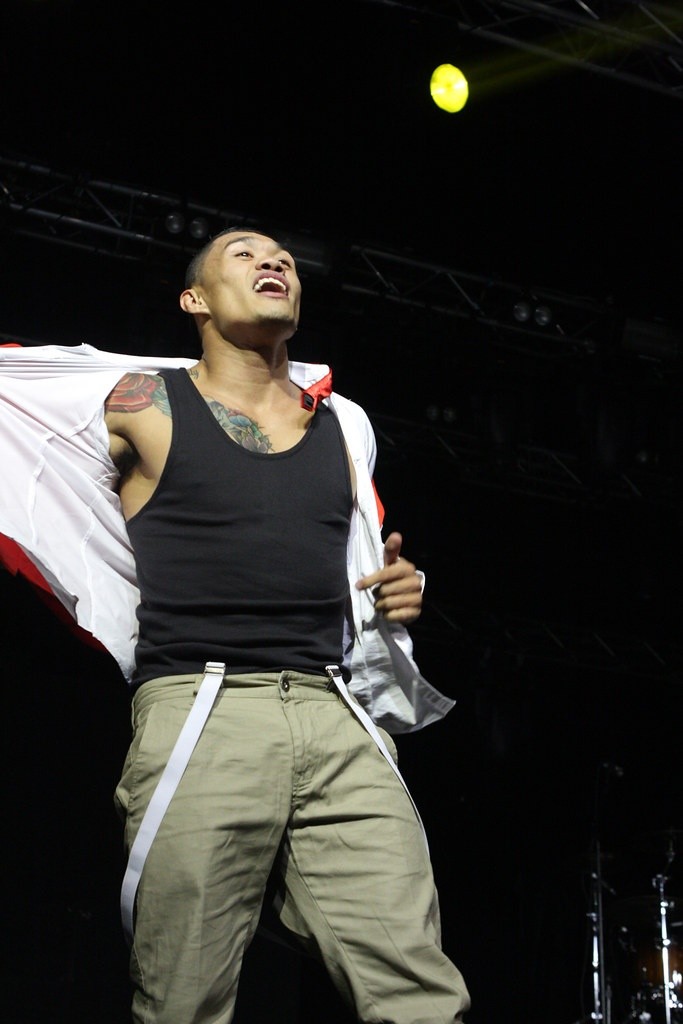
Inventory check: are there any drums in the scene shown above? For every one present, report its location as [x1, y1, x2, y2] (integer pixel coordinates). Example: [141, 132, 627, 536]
[626, 934, 683, 1003]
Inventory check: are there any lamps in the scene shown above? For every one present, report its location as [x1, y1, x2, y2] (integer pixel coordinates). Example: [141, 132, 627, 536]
[490, 287, 554, 331]
[159, 210, 210, 246]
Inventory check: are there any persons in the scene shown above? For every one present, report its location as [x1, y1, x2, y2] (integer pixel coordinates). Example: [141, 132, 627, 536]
[0, 228, 475, 1024]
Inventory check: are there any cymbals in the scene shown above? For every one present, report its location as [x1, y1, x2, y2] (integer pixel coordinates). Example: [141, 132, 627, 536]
[632, 829, 683, 854]
[609, 894, 683, 929]
